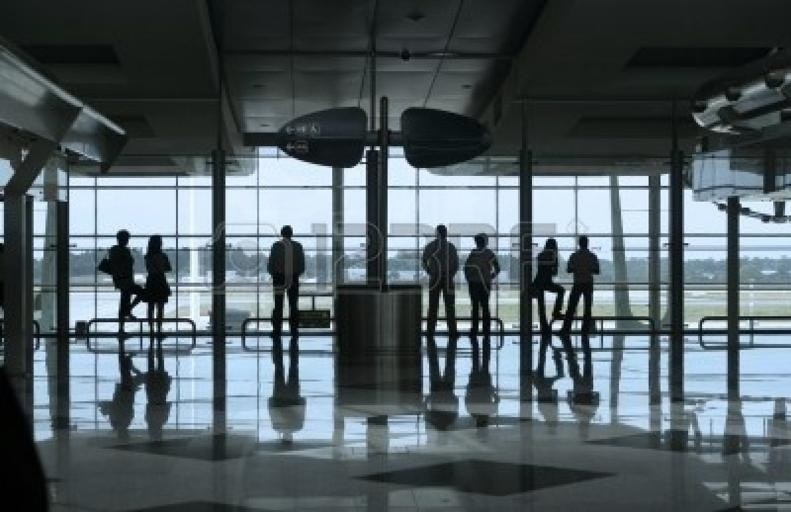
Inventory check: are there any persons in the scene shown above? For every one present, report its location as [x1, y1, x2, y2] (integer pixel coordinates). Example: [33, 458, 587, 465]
[142, 235, 173, 321]
[464, 334, 500, 427]
[533, 331, 564, 434]
[144, 321, 173, 444]
[562, 236, 599, 334]
[422, 225, 459, 336]
[97, 319, 144, 442]
[423, 336, 459, 430]
[537, 239, 565, 331]
[559, 333, 599, 442]
[464, 233, 501, 334]
[267, 225, 306, 336]
[268, 336, 306, 446]
[108, 231, 143, 321]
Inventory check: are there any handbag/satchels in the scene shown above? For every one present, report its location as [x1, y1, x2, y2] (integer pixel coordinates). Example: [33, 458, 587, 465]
[98, 249, 119, 276]
